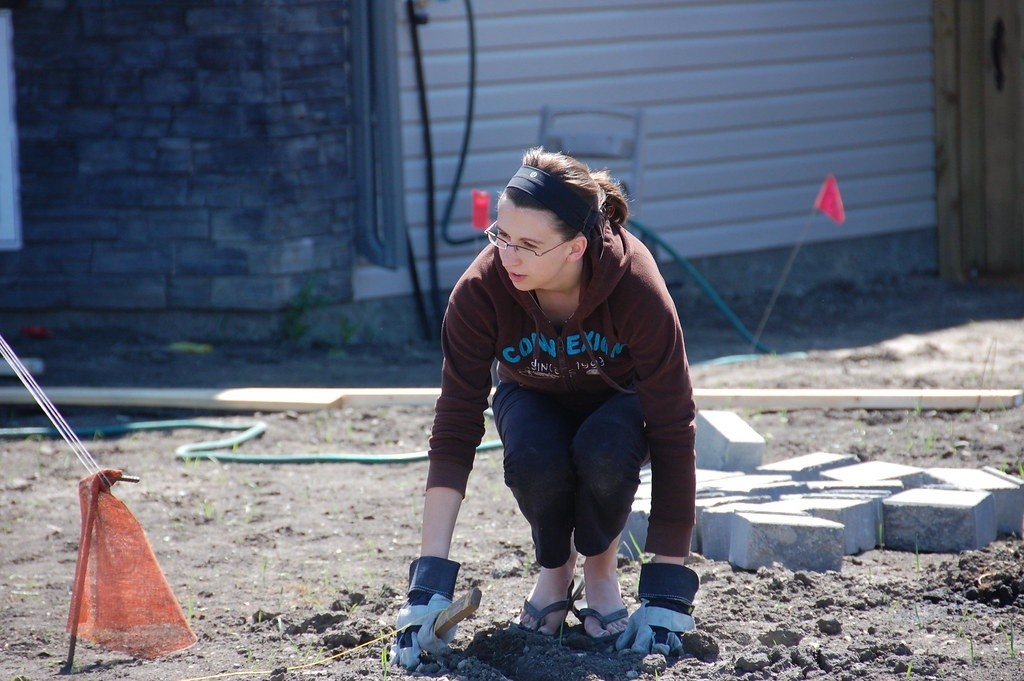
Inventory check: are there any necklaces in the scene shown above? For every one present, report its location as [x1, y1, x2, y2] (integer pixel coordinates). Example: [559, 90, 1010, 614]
[528, 291, 574, 323]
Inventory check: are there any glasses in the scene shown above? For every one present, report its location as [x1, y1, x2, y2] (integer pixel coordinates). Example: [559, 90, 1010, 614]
[484, 220, 571, 259]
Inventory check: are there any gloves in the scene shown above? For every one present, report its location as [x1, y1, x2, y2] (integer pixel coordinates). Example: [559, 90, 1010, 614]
[615, 563, 700, 660]
[389, 556, 461, 671]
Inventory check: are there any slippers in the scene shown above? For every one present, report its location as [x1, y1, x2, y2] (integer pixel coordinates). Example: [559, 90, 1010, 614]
[520, 579, 574, 639]
[578, 607, 628, 643]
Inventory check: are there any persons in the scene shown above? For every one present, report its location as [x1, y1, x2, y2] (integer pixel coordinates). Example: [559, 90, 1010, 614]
[389, 147, 699, 669]
